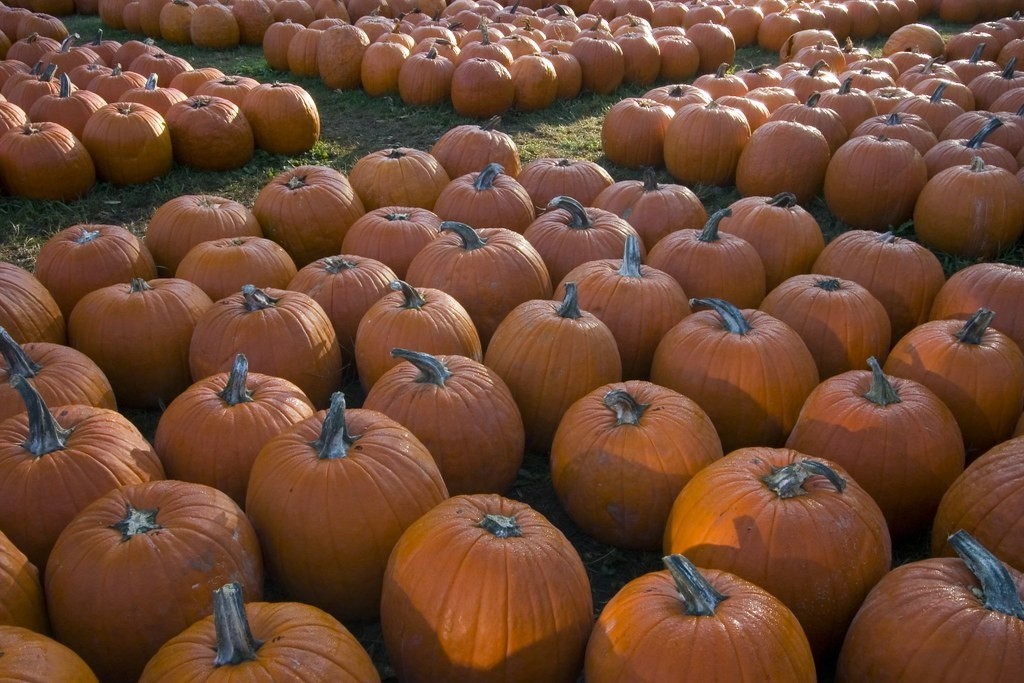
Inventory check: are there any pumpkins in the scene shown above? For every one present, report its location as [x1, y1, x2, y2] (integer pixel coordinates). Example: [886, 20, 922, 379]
[0, 113, 1024, 683]
[0, 0, 1024, 257]
